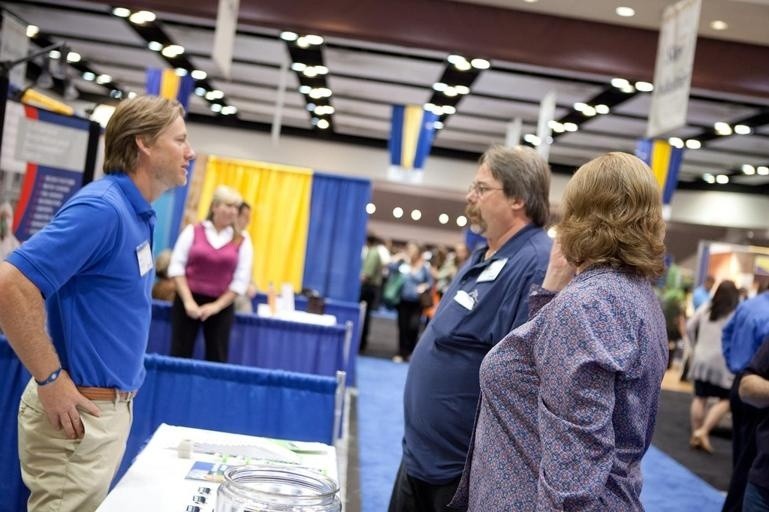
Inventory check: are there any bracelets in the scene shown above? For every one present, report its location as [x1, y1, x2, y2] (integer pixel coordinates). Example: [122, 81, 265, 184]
[34, 365, 63, 386]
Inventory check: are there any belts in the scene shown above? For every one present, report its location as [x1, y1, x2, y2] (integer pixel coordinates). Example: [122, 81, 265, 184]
[79, 387, 137, 402]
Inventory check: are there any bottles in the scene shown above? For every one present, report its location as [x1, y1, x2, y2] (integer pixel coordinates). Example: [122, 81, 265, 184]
[216, 460, 343, 511]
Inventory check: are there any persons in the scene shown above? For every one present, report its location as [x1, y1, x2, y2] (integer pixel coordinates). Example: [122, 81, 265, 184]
[358, 233, 469, 363]
[236, 202, 251, 232]
[0, 201, 21, 263]
[661, 267, 769, 512]
[167, 184, 254, 364]
[446, 152, 669, 511]
[387, 144, 554, 512]
[151, 247, 177, 303]
[0, 94, 196, 512]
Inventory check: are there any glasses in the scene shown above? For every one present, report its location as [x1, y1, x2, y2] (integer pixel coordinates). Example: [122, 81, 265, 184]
[467, 183, 504, 197]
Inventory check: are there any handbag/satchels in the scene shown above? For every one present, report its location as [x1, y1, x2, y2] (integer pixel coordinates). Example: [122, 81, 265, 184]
[383, 275, 404, 310]
[421, 288, 434, 307]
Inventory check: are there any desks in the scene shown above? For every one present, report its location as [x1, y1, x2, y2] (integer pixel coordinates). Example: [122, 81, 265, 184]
[94, 421, 343, 512]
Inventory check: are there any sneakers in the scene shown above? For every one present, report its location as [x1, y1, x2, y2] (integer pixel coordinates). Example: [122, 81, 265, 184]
[691, 429, 712, 455]
